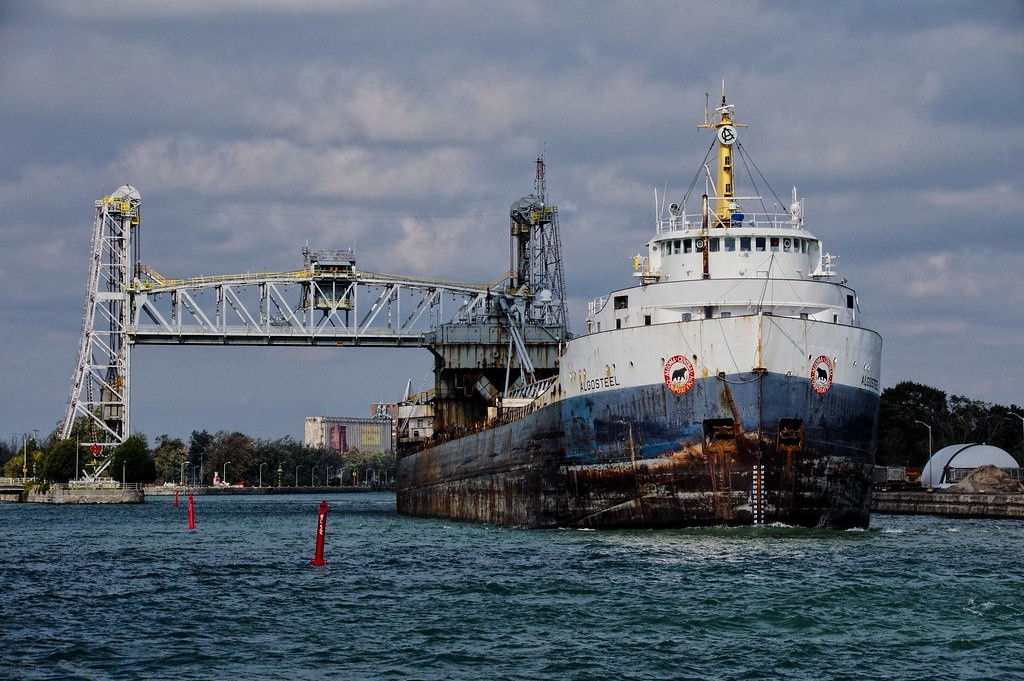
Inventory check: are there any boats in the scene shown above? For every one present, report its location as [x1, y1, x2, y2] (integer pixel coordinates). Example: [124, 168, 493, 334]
[395, 78, 882, 540]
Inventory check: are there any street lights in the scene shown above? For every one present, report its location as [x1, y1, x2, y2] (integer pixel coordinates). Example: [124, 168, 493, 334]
[259, 463, 267, 487]
[326, 466, 332, 486]
[224, 462, 230, 483]
[194, 466, 200, 486]
[295, 465, 302, 487]
[180, 461, 190, 488]
[312, 466, 318, 487]
[353, 468, 359, 486]
[915, 420, 933, 489]
[340, 467, 346, 486]
[366, 468, 387, 486]
